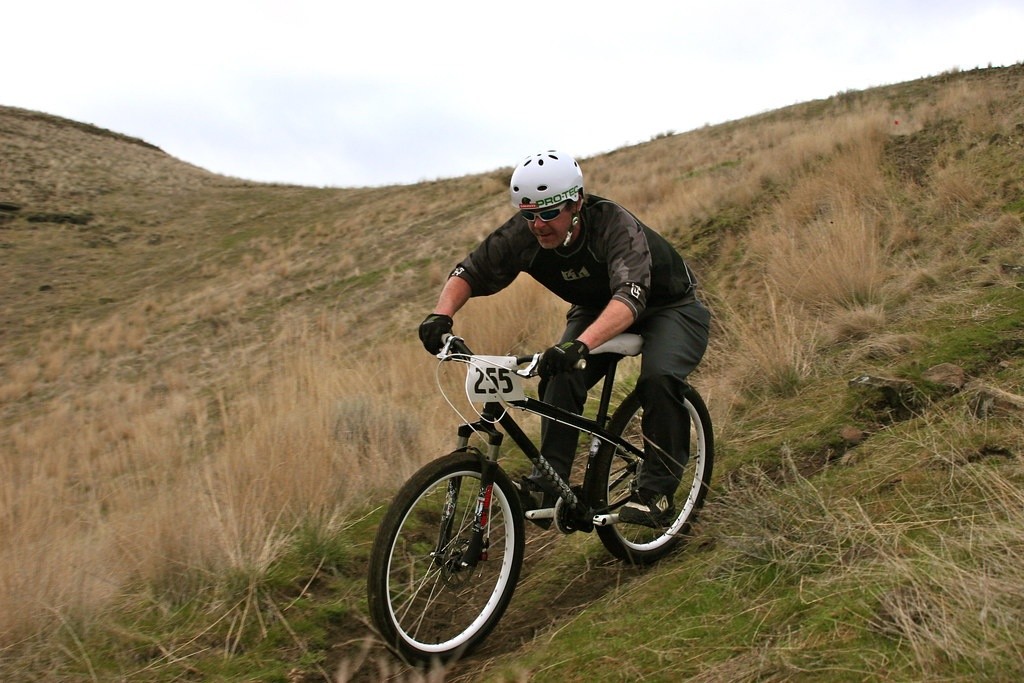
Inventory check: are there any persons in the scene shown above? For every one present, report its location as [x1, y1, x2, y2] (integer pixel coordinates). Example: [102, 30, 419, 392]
[420, 148, 711, 531]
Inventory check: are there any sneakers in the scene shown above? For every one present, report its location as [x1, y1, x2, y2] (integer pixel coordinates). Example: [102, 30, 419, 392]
[618, 489, 677, 528]
[511, 477, 557, 530]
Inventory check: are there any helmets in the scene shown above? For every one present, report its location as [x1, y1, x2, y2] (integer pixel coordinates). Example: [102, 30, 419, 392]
[509, 149, 585, 209]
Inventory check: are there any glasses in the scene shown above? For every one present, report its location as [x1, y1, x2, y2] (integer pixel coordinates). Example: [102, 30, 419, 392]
[519, 200, 569, 221]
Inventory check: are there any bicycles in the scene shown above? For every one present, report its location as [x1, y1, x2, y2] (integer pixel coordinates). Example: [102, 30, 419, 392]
[365, 326, 714, 666]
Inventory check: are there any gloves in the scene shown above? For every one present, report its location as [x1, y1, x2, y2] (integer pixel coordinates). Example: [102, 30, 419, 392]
[537, 338, 589, 382]
[418, 313, 454, 355]
